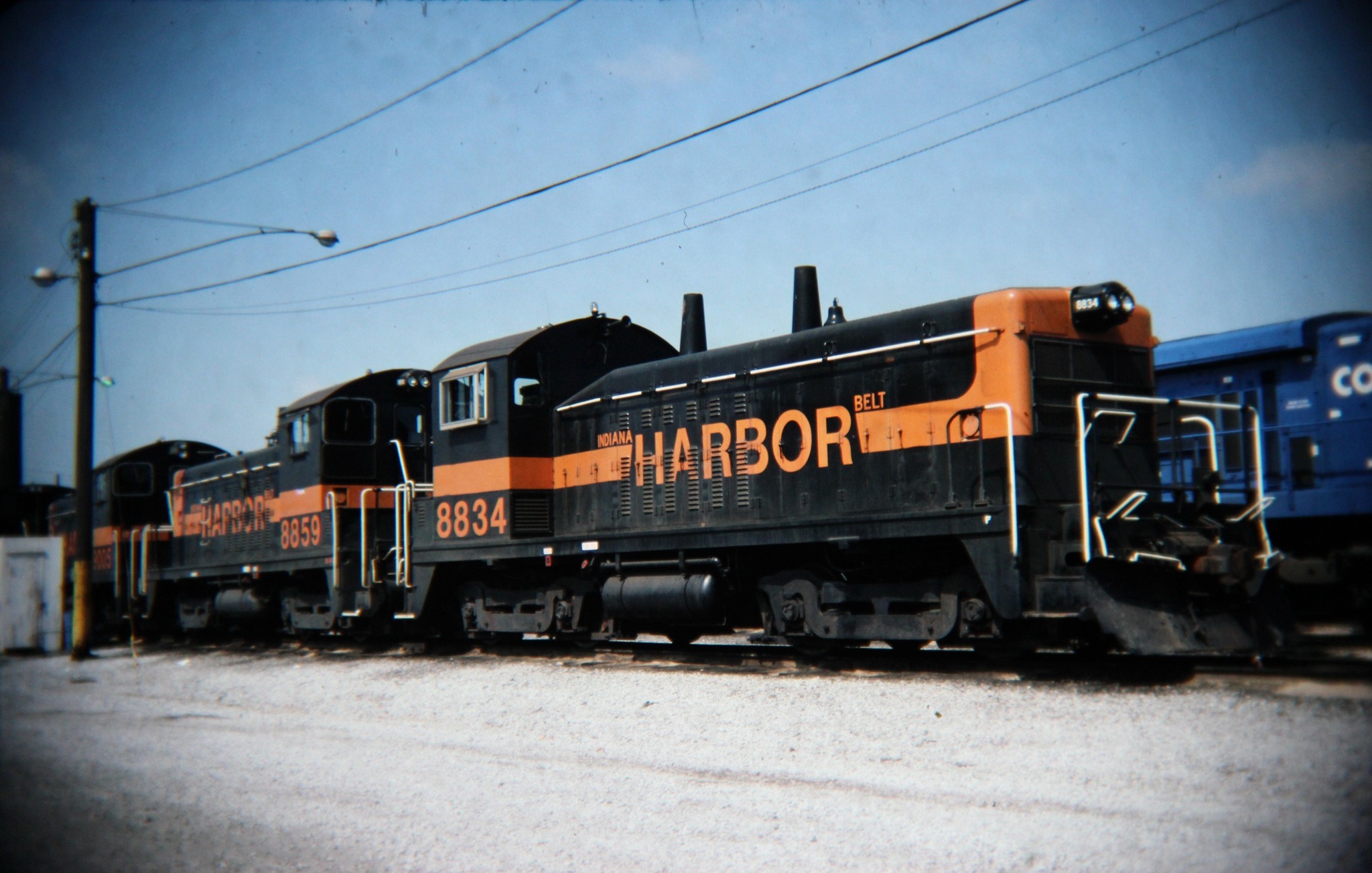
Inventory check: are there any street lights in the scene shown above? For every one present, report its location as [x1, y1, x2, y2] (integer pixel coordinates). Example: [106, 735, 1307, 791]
[31, 198, 344, 664]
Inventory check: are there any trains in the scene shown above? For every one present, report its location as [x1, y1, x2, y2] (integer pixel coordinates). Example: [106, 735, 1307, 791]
[1151, 308, 1372, 623]
[46, 264, 1307, 683]
